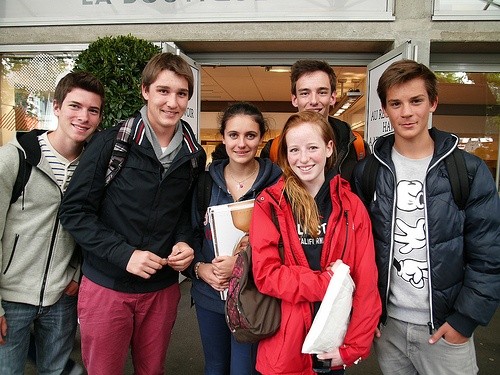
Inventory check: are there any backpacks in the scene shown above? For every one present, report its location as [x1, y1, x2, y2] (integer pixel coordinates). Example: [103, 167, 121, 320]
[224, 244, 280, 343]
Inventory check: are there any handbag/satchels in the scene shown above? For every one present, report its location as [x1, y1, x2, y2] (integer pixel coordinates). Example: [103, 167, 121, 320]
[301, 259, 361, 364]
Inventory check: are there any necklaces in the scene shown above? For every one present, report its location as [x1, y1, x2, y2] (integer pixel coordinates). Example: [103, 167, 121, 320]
[228, 160, 257, 189]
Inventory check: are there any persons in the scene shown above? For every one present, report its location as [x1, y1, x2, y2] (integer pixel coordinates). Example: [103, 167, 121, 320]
[178, 102, 284, 375]
[260, 58, 372, 186]
[0, 73, 105, 375]
[249, 108, 383, 375]
[58, 52, 208, 375]
[349, 61, 500, 374]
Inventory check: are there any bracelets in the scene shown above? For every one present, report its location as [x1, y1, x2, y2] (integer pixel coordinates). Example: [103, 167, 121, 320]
[195, 262, 204, 279]
